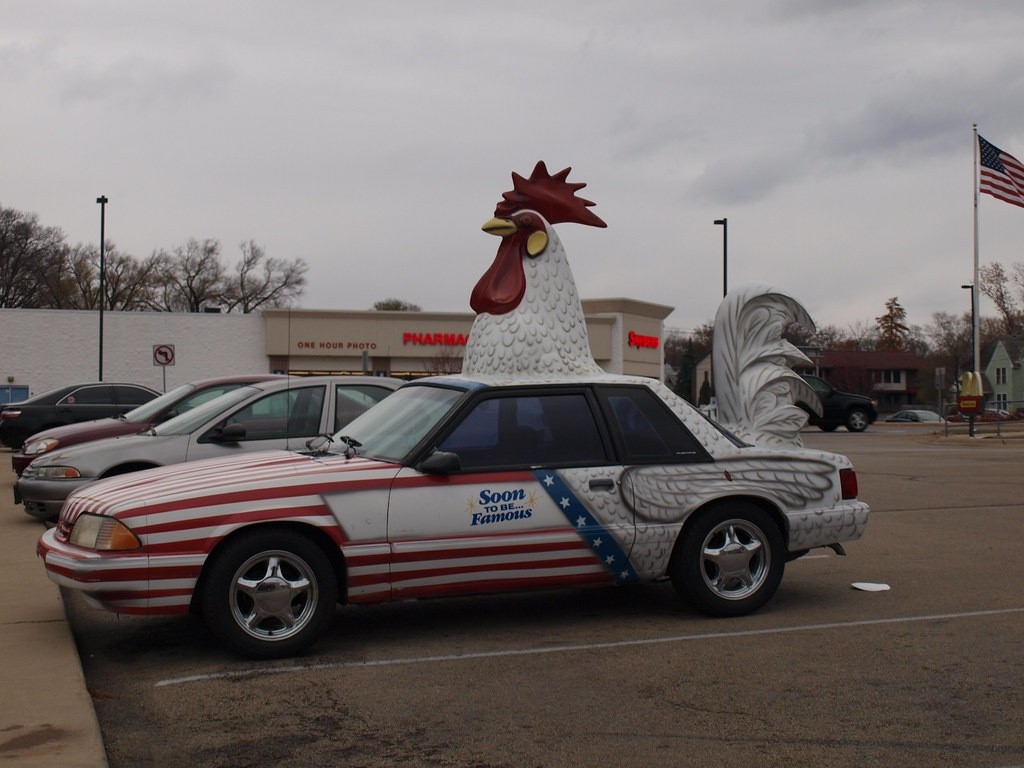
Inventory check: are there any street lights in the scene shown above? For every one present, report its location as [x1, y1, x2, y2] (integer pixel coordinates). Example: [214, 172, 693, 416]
[961, 284, 976, 372]
[95, 193, 110, 381]
[712, 217, 728, 299]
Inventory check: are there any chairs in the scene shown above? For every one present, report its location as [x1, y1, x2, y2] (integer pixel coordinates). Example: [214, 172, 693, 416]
[556, 425, 604, 461]
[483, 426, 535, 469]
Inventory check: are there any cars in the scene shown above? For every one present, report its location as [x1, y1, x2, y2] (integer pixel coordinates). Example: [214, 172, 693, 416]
[9, 374, 456, 525]
[883, 409, 947, 423]
[946, 405, 1024, 424]
[0, 380, 196, 452]
[31, 160, 871, 665]
[10, 373, 370, 476]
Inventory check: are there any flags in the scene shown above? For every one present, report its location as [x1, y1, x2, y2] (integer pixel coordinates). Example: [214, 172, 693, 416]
[978, 135, 1024, 208]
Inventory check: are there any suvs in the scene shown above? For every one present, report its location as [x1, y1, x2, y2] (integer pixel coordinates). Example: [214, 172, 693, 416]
[795, 373, 880, 433]
